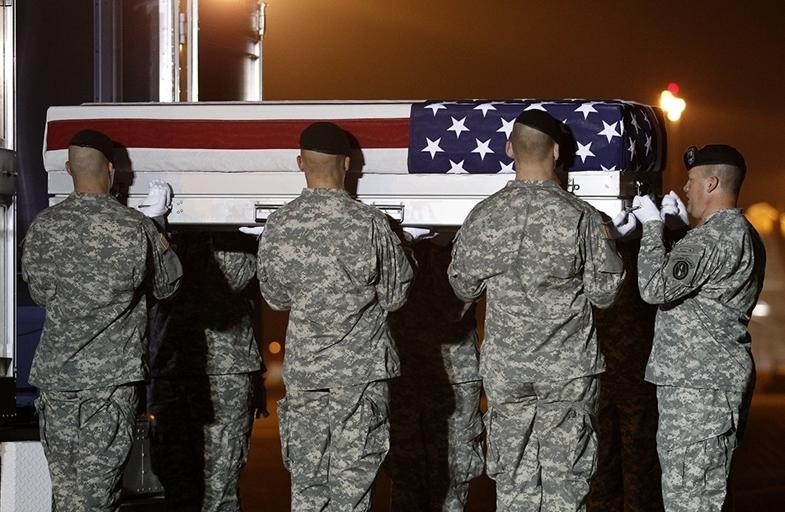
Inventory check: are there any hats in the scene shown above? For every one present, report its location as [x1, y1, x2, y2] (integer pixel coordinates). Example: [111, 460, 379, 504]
[514, 110, 573, 147]
[299, 123, 354, 157]
[69, 130, 117, 169]
[684, 144, 747, 172]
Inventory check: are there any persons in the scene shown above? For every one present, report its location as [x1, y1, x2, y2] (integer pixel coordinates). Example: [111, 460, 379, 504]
[255, 120, 421, 511]
[372, 225, 486, 511]
[147, 219, 266, 511]
[20, 129, 185, 512]
[444, 108, 637, 510]
[632, 132, 768, 511]
[584, 225, 687, 510]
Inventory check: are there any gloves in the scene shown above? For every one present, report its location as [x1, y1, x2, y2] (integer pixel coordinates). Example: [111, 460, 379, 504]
[660, 190, 690, 231]
[137, 180, 172, 219]
[608, 211, 637, 242]
[631, 194, 663, 225]
